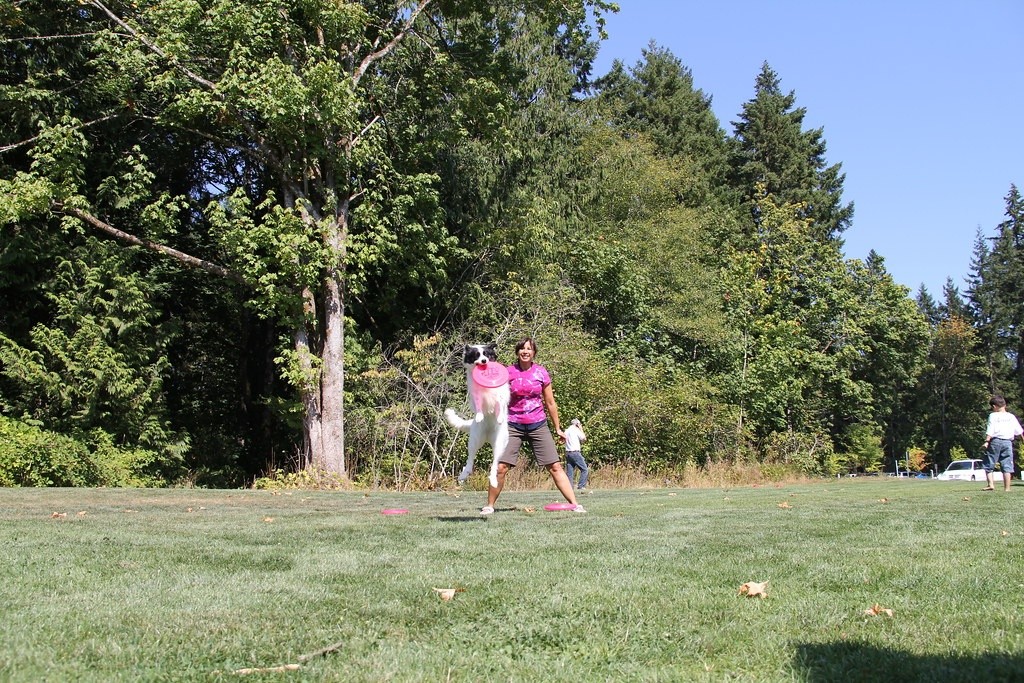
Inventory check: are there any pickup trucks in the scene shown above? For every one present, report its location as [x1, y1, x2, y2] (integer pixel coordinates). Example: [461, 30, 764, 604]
[938, 460, 1004, 482]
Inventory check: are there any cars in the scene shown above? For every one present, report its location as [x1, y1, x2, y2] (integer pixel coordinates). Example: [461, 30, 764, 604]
[888, 472, 931, 479]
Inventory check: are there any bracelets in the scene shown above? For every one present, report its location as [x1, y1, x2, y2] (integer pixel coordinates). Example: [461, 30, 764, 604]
[984, 440, 989, 443]
[556, 430, 561, 433]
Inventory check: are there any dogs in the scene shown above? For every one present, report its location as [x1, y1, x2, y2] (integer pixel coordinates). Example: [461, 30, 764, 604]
[443, 343, 511, 488]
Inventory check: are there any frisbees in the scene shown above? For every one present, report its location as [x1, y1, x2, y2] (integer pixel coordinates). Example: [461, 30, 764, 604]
[544, 502, 577, 511]
[471, 361, 511, 389]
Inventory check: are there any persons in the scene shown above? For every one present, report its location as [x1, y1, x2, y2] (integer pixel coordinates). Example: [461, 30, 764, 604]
[979, 395, 1024, 491]
[564, 418, 578, 489]
[481, 338, 586, 515]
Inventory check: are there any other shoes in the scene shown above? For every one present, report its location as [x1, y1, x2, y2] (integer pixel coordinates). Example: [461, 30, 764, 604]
[480, 506, 494, 514]
[573, 503, 586, 513]
